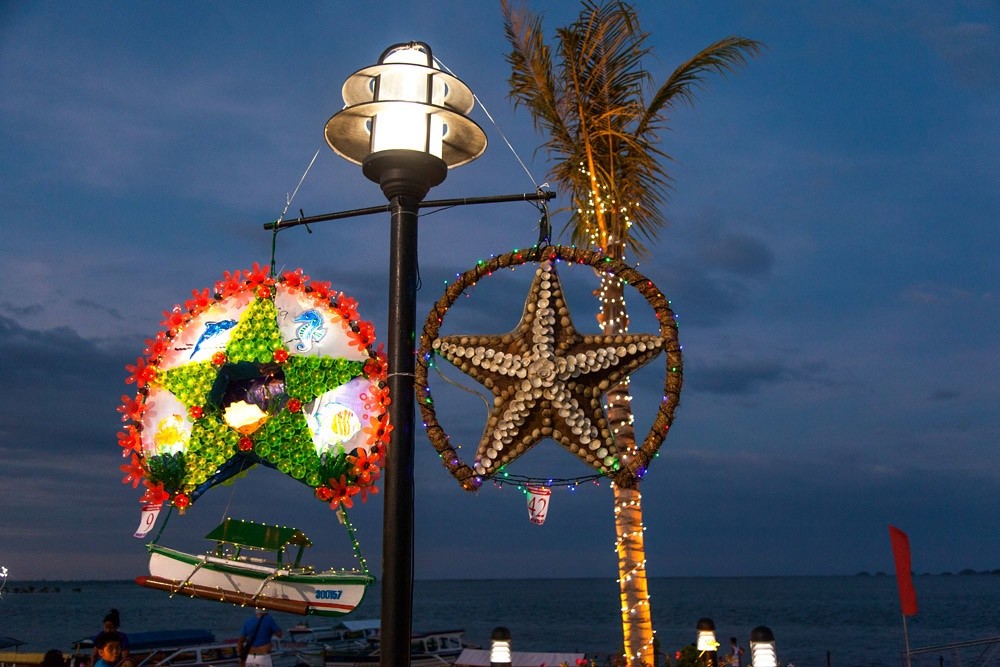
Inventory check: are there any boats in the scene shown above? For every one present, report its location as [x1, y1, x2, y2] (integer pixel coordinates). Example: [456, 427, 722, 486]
[135, 542, 378, 619]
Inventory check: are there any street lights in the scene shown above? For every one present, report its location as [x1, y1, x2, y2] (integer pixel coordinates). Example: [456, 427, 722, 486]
[263, 40, 559, 665]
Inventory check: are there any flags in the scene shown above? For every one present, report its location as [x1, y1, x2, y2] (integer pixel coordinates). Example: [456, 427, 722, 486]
[887, 524, 918, 618]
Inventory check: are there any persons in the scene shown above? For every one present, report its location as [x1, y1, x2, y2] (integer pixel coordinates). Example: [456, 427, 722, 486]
[94, 633, 121, 667]
[92, 609, 132, 666]
[238, 605, 283, 667]
[726, 637, 745, 667]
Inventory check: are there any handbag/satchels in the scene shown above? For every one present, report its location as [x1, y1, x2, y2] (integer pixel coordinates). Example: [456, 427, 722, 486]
[240, 640, 251, 660]
